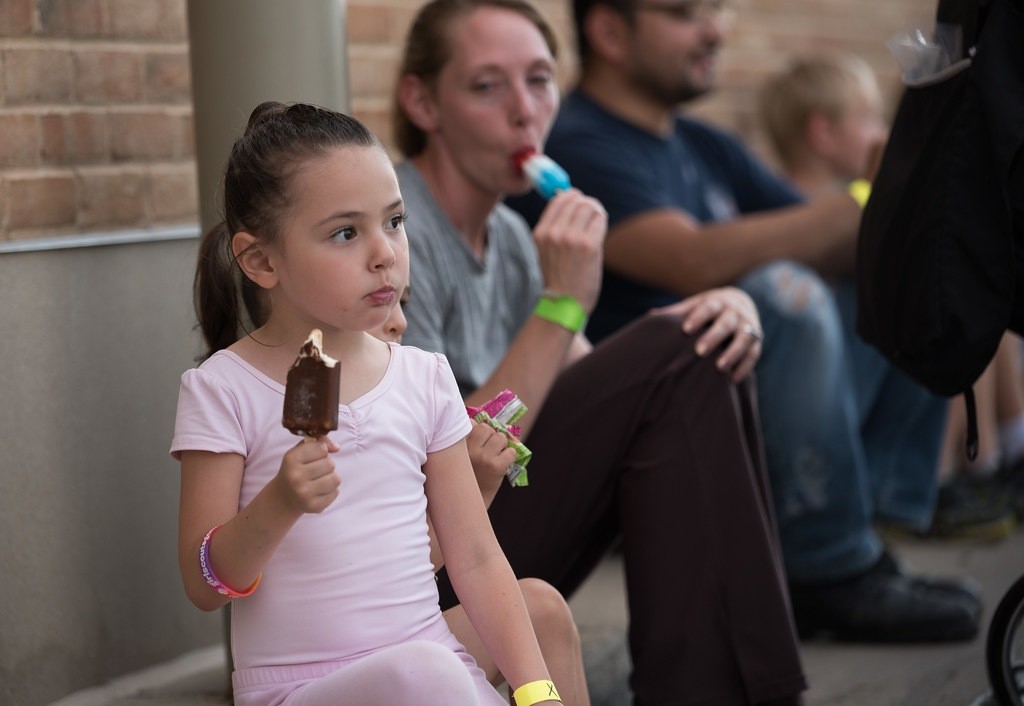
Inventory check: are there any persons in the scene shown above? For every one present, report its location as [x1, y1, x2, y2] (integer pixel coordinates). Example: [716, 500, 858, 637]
[760, 54, 1024, 539]
[167, 101, 565, 706]
[365, 267, 594, 706]
[503, 0, 985, 639]
[380, 0, 809, 706]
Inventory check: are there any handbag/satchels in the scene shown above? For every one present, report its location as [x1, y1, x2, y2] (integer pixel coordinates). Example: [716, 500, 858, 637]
[853, 0, 1021, 397]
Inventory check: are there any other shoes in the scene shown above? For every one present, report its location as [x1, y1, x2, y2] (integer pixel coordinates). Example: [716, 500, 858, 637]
[787, 554, 981, 645]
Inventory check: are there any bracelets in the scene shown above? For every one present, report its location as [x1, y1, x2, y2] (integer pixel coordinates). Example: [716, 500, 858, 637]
[535, 289, 588, 334]
[511, 680, 562, 706]
[197, 524, 263, 598]
